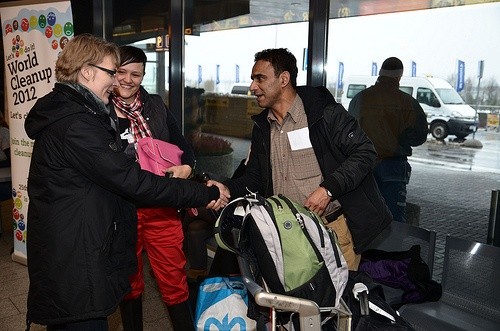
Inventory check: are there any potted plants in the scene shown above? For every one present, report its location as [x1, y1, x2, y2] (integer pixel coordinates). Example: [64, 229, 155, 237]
[192, 134, 236, 179]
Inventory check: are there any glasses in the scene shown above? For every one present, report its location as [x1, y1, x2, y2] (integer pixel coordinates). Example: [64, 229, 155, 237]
[88, 63, 117, 79]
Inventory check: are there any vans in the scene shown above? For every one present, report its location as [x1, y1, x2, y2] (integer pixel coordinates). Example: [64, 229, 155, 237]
[341, 74, 481, 141]
[216, 82, 257, 97]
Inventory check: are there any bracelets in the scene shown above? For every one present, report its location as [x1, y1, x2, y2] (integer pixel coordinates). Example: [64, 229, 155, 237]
[326, 189, 333, 198]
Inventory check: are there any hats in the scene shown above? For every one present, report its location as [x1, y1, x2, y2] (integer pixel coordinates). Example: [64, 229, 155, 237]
[379, 57, 403, 77]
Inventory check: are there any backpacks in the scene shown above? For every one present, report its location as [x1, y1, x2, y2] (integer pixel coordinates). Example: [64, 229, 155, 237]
[214, 193, 349, 331]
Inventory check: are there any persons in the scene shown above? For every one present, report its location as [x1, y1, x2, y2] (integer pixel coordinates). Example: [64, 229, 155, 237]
[25, 34, 229, 331]
[348, 57, 428, 223]
[108, 46, 197, 331]
[205, 47, 394, 271]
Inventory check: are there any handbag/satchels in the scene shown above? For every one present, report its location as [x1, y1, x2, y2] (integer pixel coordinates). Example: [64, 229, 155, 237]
[195, 277, 258, 331]
[137, 137, 184, 176]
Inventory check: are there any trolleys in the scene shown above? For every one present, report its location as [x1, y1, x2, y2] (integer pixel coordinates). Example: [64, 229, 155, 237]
[231, 221, 373, 331]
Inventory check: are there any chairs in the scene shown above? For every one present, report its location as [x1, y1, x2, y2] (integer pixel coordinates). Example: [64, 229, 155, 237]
[430, 92, 435, 106]
[421, 93, 430, 105]
[397, 236, 500, 331]
[361, 221, 437, 305]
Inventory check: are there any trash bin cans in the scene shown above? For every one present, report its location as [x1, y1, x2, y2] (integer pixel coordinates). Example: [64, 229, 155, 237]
[478, 113, 488, 129]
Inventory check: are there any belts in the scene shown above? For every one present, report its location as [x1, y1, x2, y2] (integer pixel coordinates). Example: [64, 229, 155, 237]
[321, 208, 342, 224]
